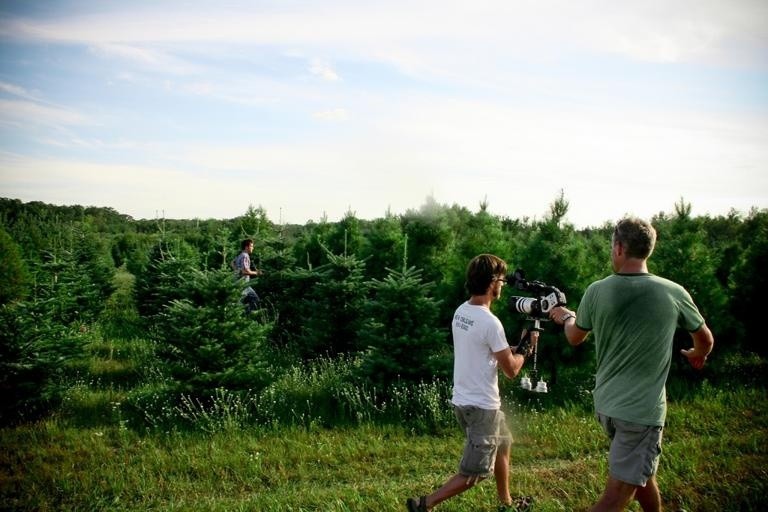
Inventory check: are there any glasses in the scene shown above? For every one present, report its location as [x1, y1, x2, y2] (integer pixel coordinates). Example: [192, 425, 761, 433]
[497, 278, 507, 285]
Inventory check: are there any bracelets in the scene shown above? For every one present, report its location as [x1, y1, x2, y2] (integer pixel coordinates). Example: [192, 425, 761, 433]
[560, 313, 576, 325]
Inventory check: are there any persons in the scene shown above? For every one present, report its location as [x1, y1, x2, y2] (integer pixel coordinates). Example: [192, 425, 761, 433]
[229, 239, 264, 317]
[544, 215, 715, 511]
[405, 251, 539, 512]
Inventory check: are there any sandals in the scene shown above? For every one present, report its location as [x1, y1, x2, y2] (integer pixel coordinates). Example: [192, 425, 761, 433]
[499, 496, 532, 512]
[407, 495, 427, 512]
